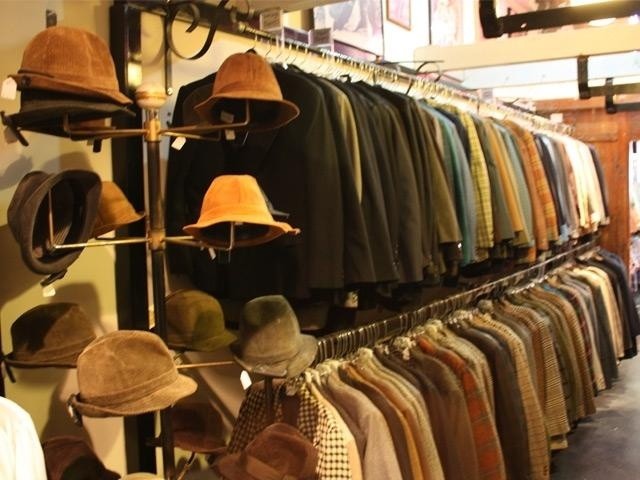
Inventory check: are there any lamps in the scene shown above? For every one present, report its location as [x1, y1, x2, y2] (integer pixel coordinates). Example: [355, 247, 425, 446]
[126, 58, 142, 93]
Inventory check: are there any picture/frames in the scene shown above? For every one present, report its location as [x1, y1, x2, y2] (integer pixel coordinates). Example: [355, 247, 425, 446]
[386, 0, 411, 31]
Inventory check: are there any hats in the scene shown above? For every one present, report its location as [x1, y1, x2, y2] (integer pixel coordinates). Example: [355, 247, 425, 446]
[41, 435, 121, 480]
[7, 169, 147, 274]
[0, 25, 136, 125]
[181, 174, 301, 248]
[192, 52, 301, 132]
[145, 401, 319, 480]
